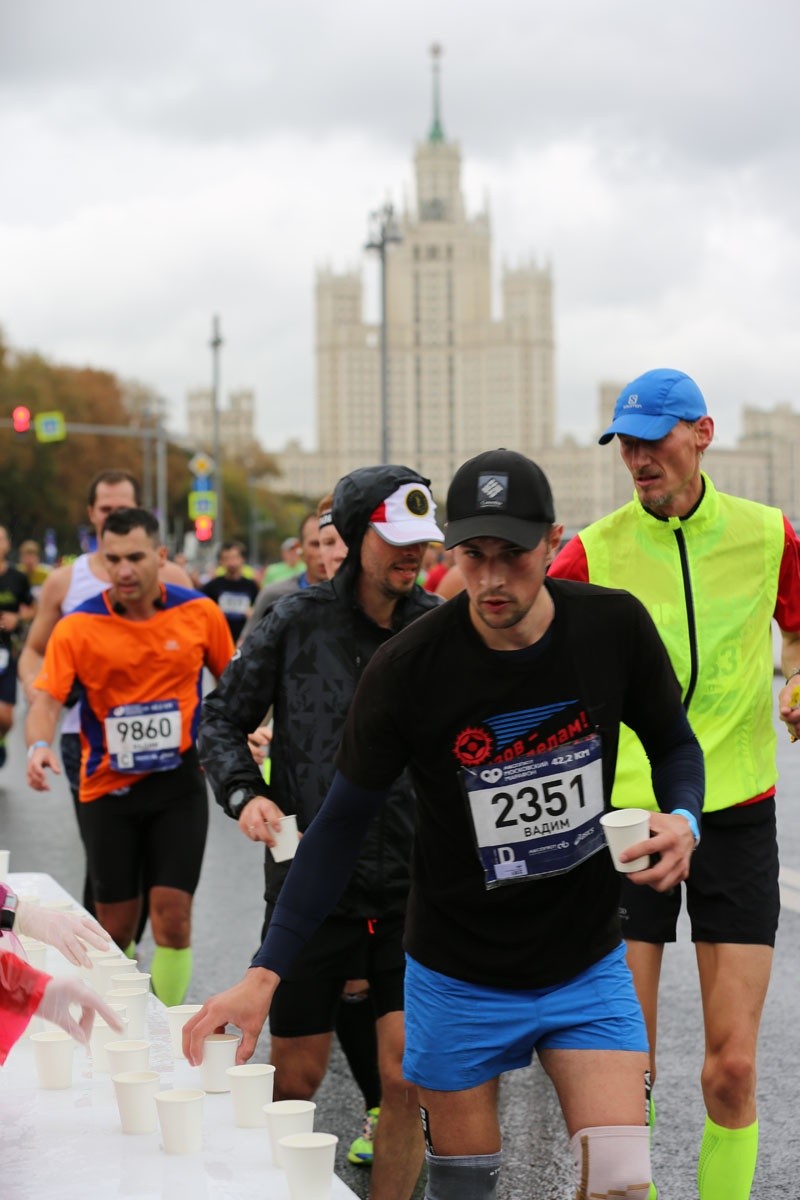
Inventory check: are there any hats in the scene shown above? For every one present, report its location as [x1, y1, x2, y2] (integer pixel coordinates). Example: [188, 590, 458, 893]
[368, 483, 445, 546]
[598, 368, 707, 445]
[444, 448, 555, 552]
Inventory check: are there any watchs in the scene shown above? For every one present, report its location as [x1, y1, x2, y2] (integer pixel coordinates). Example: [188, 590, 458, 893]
[228, 788, 258, 820]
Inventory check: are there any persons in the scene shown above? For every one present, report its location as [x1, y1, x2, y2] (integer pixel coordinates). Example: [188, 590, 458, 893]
[180, 448, 705, 1200]
[548, 368, 800, 1200]
[0, 469, 558, 1199]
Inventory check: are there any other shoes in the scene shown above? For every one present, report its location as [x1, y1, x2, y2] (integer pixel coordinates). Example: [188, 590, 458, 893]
[346, 1106, 381, 1164]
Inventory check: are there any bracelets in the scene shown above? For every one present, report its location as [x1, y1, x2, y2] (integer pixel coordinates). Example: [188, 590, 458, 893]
[786, 667, 799, 684]
[669, 809, 701, 848]
[28, 742, 49, 757]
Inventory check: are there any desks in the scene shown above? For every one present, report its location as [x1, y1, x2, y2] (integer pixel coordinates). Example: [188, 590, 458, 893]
[1, 873, 359, 1200]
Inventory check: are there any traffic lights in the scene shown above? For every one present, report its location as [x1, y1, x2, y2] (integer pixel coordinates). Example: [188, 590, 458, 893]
[13, 407, 32, 449]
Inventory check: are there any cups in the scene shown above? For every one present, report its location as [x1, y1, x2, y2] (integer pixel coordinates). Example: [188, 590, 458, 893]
[228, 1063, 276, 1126]
[264, 815, 300, 863]
[95, 1004, 127, 1018]
[85, 951, 122, 989]
[167, 1004, 205, 1059]
[202, 1034, 240, 1093]
[155, 1089, 207, 1153]
[97, 959, 138, 996]
[31, 1031, 74, 1089]
[0, 850, 10, 883]
[263, 1100, 317, 1166]
[278, 1133, 339, 1200]
[21, 944, 48, 972]
[111, 973, 151, 993]
[600, 808, 651, 873]
[18, 895, 87, 943]
[104, 1040, 150, 1072]
[107, 988, 148, 1026]
[112, 1070, 161, 1135]
[90, 1017, 130, 1071]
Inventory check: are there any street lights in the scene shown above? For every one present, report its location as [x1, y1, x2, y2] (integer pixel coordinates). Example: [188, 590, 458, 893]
[359, 215, 407, 472]
